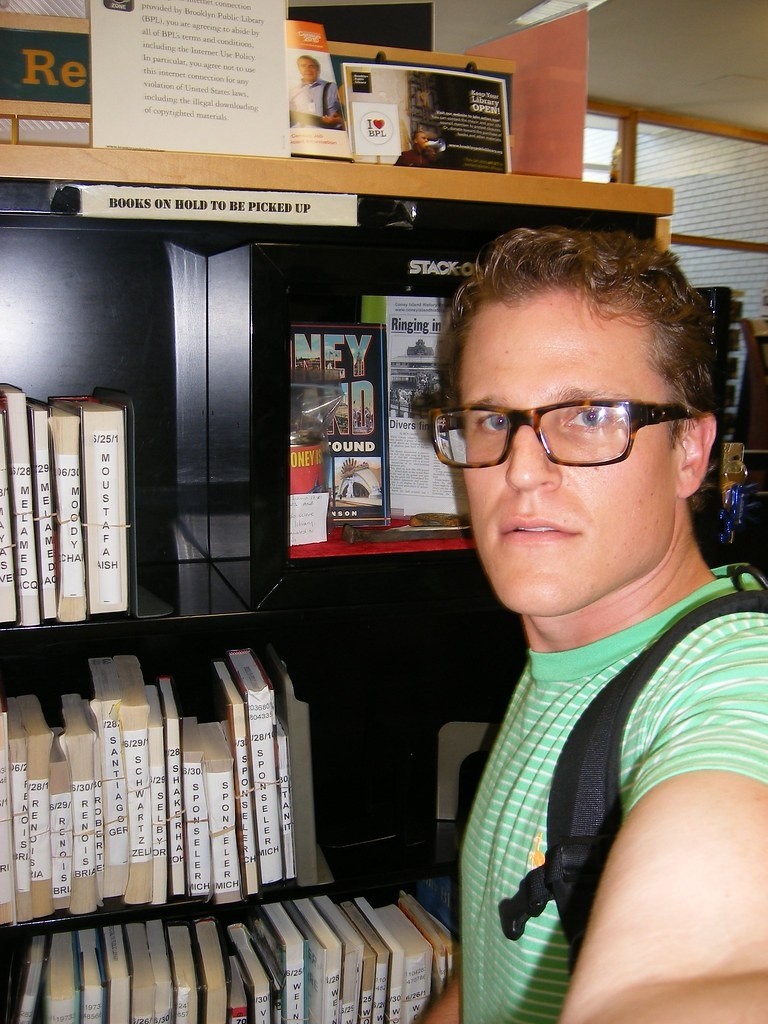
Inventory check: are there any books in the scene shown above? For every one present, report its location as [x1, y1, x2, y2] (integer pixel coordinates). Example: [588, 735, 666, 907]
[0, 0, 510, 1024]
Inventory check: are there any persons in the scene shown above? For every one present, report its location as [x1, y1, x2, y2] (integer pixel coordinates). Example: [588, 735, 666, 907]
[408, 226, 768, 1023]
[291, 55, 346, 130]
[394, 129, 442, 170]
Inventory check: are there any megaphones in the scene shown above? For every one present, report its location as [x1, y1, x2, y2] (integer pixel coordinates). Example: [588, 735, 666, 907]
[423, 138, 447, 152]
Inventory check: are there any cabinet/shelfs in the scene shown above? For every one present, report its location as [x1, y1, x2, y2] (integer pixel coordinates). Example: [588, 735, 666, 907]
[0, 139, 676, 1024]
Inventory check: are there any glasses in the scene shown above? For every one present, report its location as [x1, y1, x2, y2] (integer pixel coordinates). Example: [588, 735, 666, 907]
[428, 400, 694, 467]
